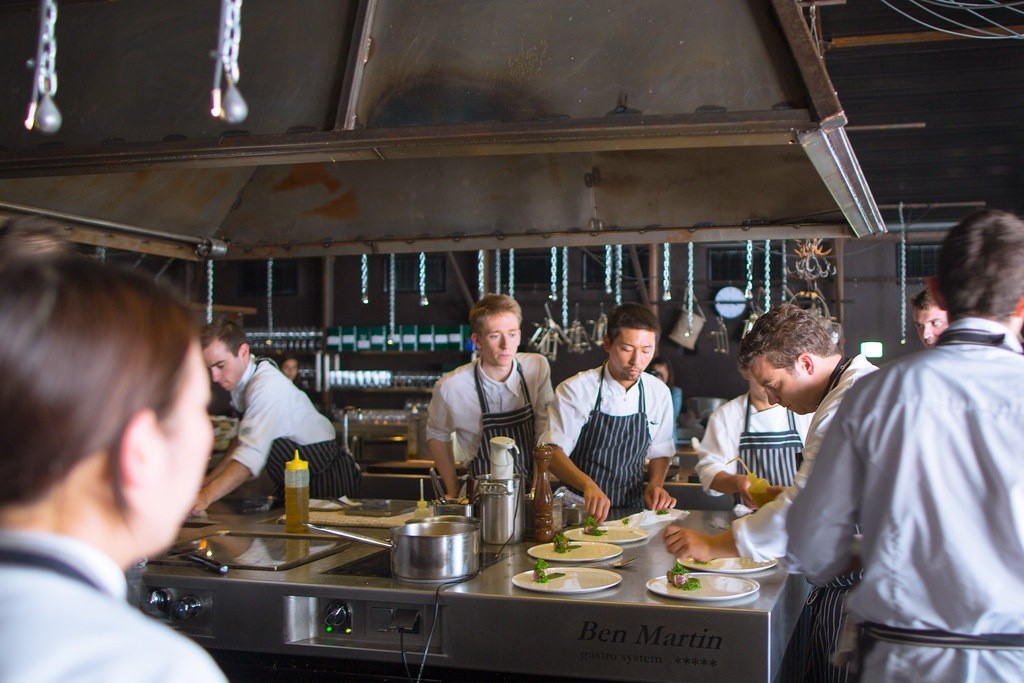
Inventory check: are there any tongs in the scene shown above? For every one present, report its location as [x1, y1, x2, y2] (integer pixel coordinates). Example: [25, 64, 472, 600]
[527, 302, 610, 362]
[429, 466, 447, 505]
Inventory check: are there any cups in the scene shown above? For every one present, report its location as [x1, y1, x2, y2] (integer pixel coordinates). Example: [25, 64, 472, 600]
[327, 368, 450, 427]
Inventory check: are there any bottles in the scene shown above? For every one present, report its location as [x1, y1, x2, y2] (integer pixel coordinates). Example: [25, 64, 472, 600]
[243, 326, 325, 352]
[284, 449, 311, 535]
[727, 458, 777, 510]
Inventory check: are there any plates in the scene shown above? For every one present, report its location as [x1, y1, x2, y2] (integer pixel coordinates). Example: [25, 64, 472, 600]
[511, 566, 623, 595]
[675, 555, 779, 574]
[345, 498, 419, 517]
[527, 540, 624, 562]
[563, 525, 648, 544]
[647, 571, 761, 602]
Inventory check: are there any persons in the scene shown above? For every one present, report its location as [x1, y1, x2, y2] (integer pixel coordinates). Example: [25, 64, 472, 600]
[647, 356, 683, 451]
[695, 341, 819, 511]
[535, 302, 680, 528]
[423, 294, 555, 502]
[181, 317, 368, 521]
[911, 288, 950, 350]
[661, 302, 883, 683]
[784, 211, 1023, 683]
[0, 270, 235, 683]
[279, 353, 303, 382]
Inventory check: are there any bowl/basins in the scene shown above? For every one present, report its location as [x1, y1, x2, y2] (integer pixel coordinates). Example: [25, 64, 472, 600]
[523, 493, 564, 534]
[685, 397, 730, 420]
[563, 503, 590, 526]
[433, 498, 475, 518]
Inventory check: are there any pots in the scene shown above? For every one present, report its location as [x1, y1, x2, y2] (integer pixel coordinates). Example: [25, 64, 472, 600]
[475, 473, 527, 545]
[298, 519, 483, 581]
[405, 513, 483, 562]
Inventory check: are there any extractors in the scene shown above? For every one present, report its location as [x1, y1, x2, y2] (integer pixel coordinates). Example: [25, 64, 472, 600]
[1, 0, 888, 262]
